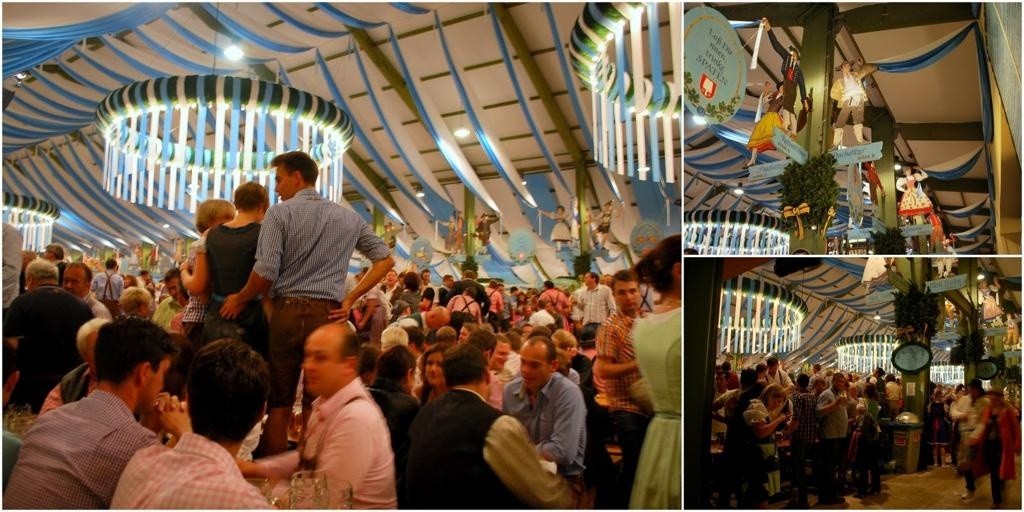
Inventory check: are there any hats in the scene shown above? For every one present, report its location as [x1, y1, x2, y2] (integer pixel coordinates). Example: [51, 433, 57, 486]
[987, 384, 1006, 398]
[579, 330, 595, 347]
[967, 379, 986, 393]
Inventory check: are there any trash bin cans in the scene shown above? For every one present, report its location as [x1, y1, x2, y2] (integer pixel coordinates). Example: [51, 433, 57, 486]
[888, 420, 924, 474]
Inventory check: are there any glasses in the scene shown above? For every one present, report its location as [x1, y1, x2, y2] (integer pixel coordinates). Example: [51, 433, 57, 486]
[24, 283, 31, 290]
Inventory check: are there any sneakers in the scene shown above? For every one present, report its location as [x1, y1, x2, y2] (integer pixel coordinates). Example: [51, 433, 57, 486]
[962, 493, 976, 503]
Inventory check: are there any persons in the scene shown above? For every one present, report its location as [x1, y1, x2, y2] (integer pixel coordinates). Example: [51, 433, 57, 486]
[829, 56, 878, 151]
[710, 355, 1021, 508]
[740, 80, 786, 170]
[864, 160, 886, 219]
[759, 17, 808, 141]
[587, 198, 622, 247]
[221, 152, 393, 456]
[434, 209, 503, 255]
[860, 257, 898, 296]
[924, 207, 946, 255]
[1003, 311, 1021, 350]
[895, 167, 933, 227]
[977, 275, 1003, 327]
[1, 222, 680, 508]
[937, 233, 958, 256]
[182, 199, 234, 347]
[179, 180, 269, 365]
[944, 297, 961, 327]
[537, 204, 573, 253]
[572, 194, 607, 251]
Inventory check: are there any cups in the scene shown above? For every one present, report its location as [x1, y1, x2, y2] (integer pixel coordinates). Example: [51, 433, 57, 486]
[248, 463, 356, 511]
